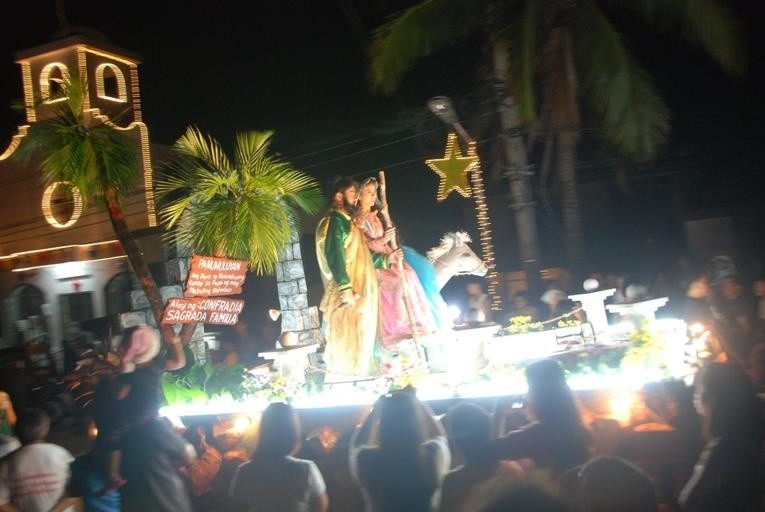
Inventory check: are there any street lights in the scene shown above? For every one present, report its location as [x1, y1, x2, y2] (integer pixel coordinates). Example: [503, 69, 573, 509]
[423, 92, 508, 315]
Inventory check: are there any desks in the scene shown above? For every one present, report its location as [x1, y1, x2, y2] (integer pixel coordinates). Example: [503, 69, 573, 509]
[568, 288, 619, 333]
[257, 342, 319, 389]
[604, 297, 669, 330]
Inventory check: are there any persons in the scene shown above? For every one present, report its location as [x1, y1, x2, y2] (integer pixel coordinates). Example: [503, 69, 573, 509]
[2, 268, 765, 512]
[352, 171, 436, 348]
[315, 175, 380, 392]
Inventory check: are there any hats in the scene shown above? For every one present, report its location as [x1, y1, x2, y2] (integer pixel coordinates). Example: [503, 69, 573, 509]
[119, 325, 160, 374]
